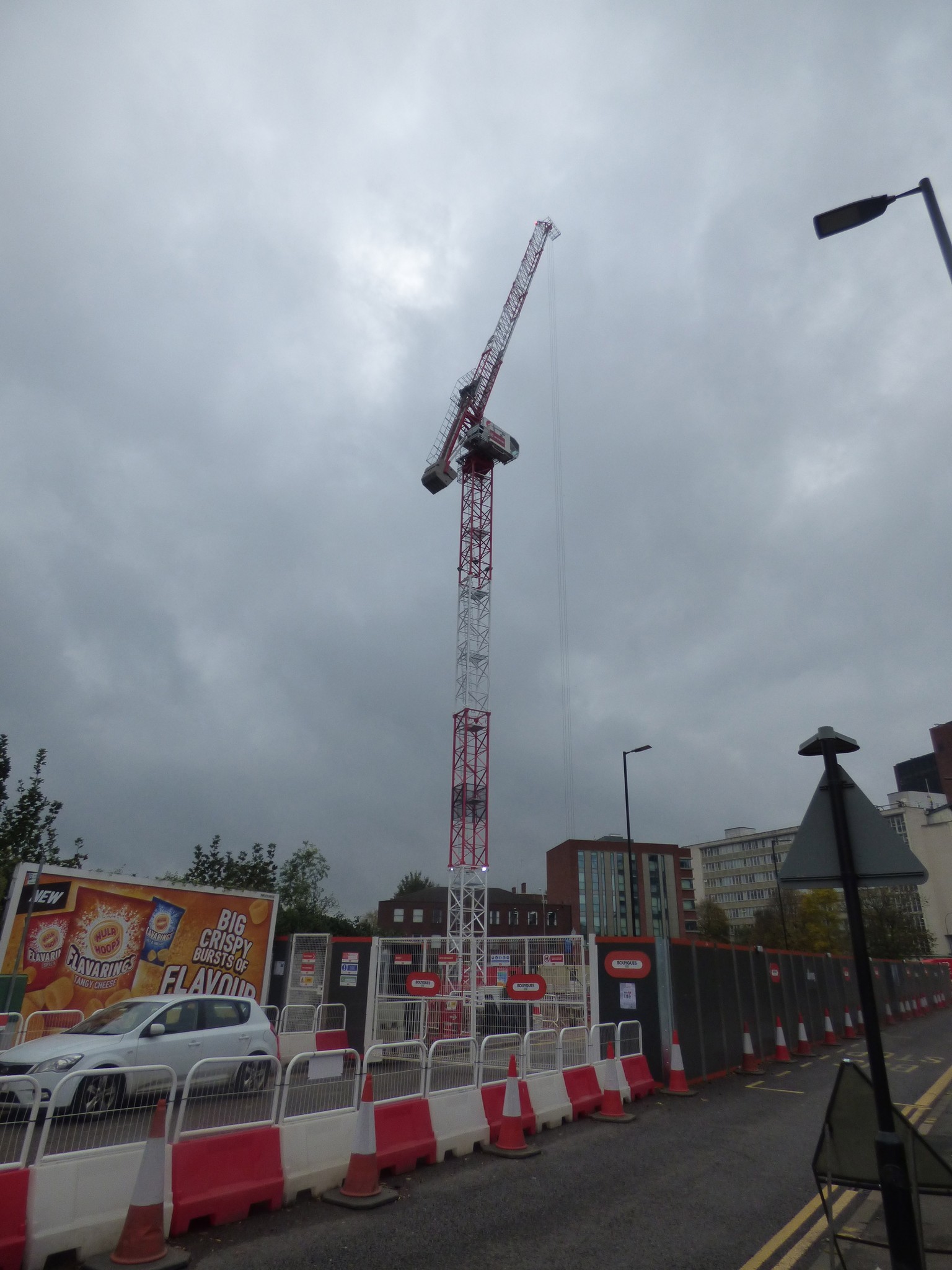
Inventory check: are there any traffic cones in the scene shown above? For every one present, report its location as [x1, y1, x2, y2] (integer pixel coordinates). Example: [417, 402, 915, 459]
[480, 1054, 544, 1160]
[659, 1030, 700, 1097]
[735, 1022, 771, 1076]
[80, 1099, 191, 1270]
[589, 1041, 637, 1123]
[318, 1074, 400, 1210]
[842, 990, 948, 1041]
[771, 1015, 796, 1063]
[821, 1007, 843, 1046]
[793, 1012, 818, 1057]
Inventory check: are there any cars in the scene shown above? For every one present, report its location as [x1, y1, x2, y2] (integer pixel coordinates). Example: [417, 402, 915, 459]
[0, 994, 280, 1120]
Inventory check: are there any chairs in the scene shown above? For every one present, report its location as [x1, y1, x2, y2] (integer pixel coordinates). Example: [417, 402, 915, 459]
[147, 1008, 167, 1029]
[176, 1007, 196, 1033]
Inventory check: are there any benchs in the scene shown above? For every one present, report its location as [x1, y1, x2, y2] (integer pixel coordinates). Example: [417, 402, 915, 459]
[204, 1012, 240, 1030]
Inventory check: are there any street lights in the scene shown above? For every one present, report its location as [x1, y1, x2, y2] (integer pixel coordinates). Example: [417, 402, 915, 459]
[770, 839, 794, 950]
[817, 176, 951, 278]
[622, 744, 654, 939]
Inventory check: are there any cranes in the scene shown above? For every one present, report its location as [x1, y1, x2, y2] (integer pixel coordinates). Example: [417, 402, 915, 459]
[422, 213, 561, 1000]
